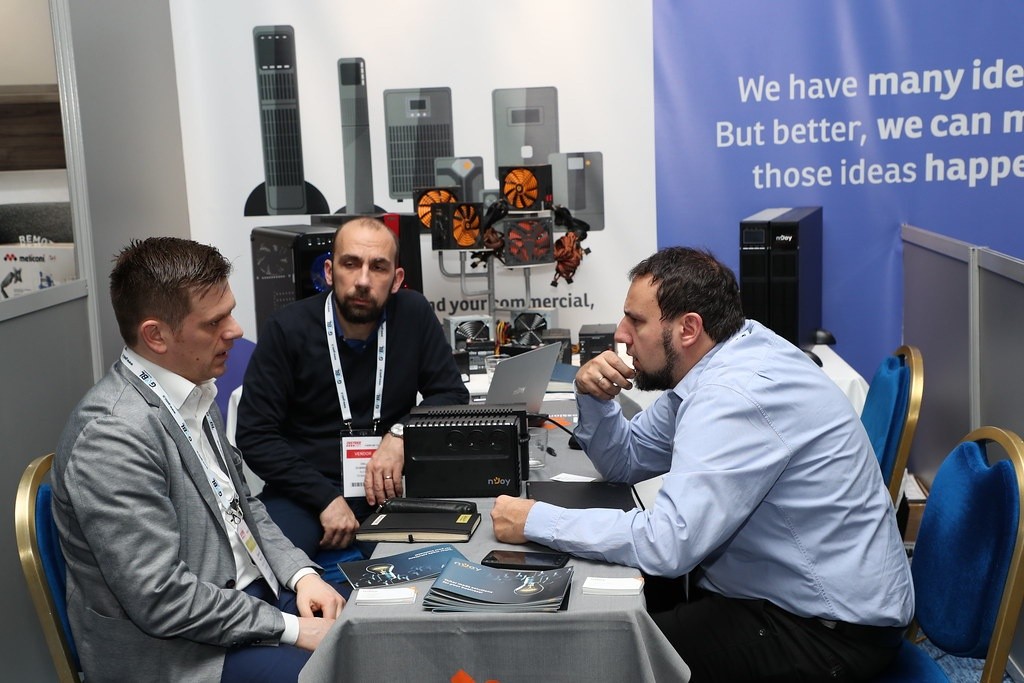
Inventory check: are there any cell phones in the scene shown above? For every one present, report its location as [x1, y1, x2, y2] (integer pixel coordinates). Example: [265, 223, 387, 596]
[481, 550, 570, 570]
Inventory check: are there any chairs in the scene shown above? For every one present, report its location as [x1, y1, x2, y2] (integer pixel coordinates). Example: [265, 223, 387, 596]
[214, 338, 256, 433]
[885, 426, 1024, 683]
[15, 453, 82, 683]
[849, 345, 924, 504]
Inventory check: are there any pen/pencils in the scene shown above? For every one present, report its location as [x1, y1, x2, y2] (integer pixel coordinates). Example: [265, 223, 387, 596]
[547, 446, 556, 457]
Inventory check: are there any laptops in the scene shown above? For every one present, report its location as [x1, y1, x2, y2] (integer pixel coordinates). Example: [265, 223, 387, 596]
[484, 341, 562, 413]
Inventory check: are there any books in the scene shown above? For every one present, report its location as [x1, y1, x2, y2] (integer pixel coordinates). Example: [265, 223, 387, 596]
[336, 544, 574, 613]
[355, 512, 481, 543]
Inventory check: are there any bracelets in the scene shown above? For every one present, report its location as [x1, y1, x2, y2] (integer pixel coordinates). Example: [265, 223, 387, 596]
[387, 429, 404, 442]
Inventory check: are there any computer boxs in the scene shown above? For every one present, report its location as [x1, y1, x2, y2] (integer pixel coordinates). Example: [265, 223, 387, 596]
[740, 205, 822, 347]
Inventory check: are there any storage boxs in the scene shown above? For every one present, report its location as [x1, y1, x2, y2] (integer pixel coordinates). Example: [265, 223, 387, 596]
[0, 243, 76, 300]
[541, 329, 571, 364]
[579, 325, 617, 366]
[467, 342, 495, 374]
[452, 351, 470, 382]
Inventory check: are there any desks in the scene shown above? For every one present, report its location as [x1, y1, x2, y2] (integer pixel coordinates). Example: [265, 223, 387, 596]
[416, 344, 869, 418]
[298, 392, 690, 683]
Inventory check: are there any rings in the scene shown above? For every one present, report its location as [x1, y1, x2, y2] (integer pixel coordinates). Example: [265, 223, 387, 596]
[385, 476, 392, 478]
[594, 376, 604, 384]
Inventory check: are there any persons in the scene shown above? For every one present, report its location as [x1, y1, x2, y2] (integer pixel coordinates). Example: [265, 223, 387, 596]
[490, 246, 916, 683]
[235, 217, 470, 561]
[49, 237, 346, 683]
